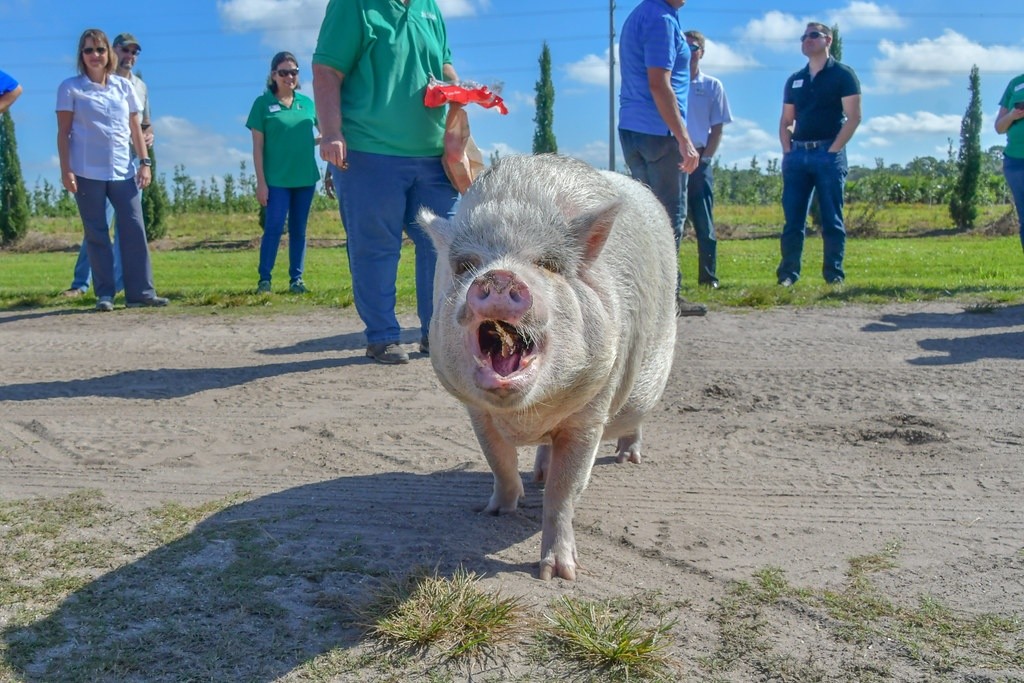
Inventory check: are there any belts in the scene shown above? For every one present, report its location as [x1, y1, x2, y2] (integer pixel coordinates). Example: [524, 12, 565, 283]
[792, 141, 818, 150]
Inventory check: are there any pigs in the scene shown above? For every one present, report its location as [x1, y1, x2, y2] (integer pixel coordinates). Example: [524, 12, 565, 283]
[415, 151, 681, 580]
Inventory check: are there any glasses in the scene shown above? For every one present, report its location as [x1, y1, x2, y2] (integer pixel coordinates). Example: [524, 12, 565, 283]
[801, 32, 826, 41]
[689, 45, 701, 52]
[278, 68, 299, 77]
[121, 47, 137, 55]
[83, 47, 107, 54]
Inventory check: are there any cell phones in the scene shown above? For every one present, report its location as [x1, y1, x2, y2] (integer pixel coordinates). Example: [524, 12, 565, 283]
[140, 123, 151, 131]
[1014, 101, 1024, 109]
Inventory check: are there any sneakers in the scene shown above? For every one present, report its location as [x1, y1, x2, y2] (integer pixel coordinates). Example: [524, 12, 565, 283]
[255, 282, 271, 296]
[292, 282, 312, 294]
[366, 343, 410, 364]
[125, 296, 170, 308]
[96, 295, 115, 312]
[420, 344, 429, 354]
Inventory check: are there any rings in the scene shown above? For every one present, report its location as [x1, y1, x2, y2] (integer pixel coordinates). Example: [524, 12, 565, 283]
[71, 181, 75, 185]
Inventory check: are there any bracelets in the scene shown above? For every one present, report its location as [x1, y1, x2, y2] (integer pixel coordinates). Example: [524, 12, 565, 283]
[325, 177, 331, 182]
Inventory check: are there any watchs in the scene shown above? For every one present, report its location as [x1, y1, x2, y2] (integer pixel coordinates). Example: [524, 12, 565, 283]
[701, 157, 712, 165]
[140, 157, 152, 166]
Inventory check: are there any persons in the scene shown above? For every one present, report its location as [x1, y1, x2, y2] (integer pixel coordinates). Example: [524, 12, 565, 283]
[55, 29, 169, 311]
[683, 30, 733, 288]
[995, 74, 1024, 253]
[324, 161, 337, 199]
[245, 52, 322, 294]
[776, 22, 861, 287]
[0, 70, 23, 114]
[618, 0, 707, 318]
[59, 33, 154, 298]
[311, 0, 468, 364]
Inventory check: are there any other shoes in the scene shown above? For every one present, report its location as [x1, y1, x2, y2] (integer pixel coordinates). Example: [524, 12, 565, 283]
[61, 288, 83, 299]
[677, 297, 708, 316]
[778, 277, 794, 289]
